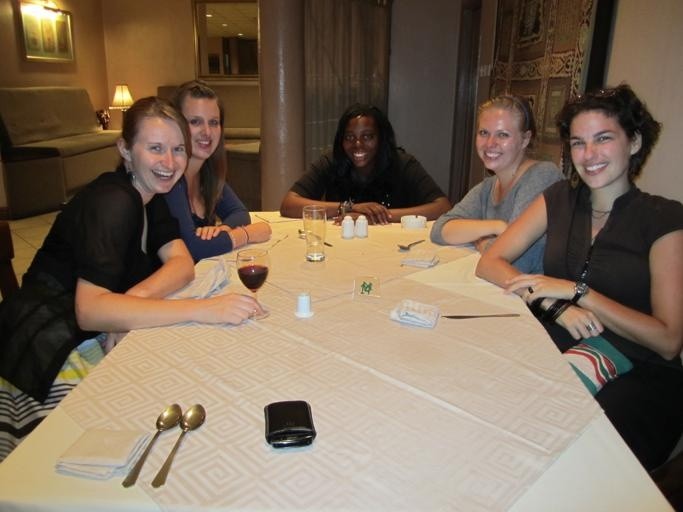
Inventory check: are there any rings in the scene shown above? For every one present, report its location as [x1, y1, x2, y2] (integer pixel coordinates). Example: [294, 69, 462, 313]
[588, 324, 595, 330]
[528, 286, 534, 294]
[543, 300, 570, 323]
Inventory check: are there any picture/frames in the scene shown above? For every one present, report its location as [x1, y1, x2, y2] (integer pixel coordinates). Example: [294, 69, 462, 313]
[14, 0, 76, 65]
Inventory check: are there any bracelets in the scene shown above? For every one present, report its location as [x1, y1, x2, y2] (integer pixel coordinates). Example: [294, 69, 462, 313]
[336, 199, 353, 215]
[238, 227, 249, 245]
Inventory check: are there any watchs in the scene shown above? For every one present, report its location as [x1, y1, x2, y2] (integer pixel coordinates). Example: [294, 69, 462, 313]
[571, 281, 589, 305]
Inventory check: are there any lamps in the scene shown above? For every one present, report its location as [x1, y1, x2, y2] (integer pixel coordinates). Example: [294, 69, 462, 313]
[109, 84, 134, 131]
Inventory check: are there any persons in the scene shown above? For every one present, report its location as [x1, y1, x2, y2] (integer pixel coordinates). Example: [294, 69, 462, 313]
[163, 78, 272, 260]
[279, 102, 453, 223]
[430, 91, 565, 276]
[475, 87, 683, 469]
[0, 97, 264, 458]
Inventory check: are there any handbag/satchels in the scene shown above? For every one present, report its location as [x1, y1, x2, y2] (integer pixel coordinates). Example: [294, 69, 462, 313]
[561, 333, 634, 397]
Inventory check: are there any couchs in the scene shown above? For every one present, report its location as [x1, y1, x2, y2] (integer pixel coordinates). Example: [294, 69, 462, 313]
[157, 82, 261, 210]
[0, 87, 122, 221]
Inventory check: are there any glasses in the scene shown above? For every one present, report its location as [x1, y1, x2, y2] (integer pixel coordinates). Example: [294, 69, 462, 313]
[573, 87, 617, 104]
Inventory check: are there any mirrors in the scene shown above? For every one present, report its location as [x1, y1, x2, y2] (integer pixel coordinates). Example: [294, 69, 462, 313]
[191, 0, 261, 86]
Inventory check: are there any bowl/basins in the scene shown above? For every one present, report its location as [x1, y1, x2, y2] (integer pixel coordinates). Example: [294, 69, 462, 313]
[400, 215, 426, 232]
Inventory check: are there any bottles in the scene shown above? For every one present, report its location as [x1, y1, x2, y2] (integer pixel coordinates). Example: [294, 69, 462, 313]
[356, 216, 368, 237]
[296, 291, 310, 315]
[342, 216, 354, 239]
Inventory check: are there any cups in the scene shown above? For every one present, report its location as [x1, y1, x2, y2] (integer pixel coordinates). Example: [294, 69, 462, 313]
[237, 250, 269, 319]
[351, 276, 382, 304]
[304, 205, 327, 262]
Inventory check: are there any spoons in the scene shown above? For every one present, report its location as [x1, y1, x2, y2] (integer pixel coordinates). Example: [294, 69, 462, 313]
[396, 240, 426, 251]
[150, 404, 209, 487]
[122, 405, 181, 487]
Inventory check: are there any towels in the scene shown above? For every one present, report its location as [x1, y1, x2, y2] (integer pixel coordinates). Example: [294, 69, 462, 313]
[389, 298, 440, 329]
[162, 254, 229, 300]
[399, 254, 440, 268]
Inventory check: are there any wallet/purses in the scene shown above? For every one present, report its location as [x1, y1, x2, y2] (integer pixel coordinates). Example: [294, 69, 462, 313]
[262, 399, 317, 448]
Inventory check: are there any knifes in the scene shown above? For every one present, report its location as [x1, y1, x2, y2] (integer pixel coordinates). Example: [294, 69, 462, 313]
[442, 313, 519, 321]
